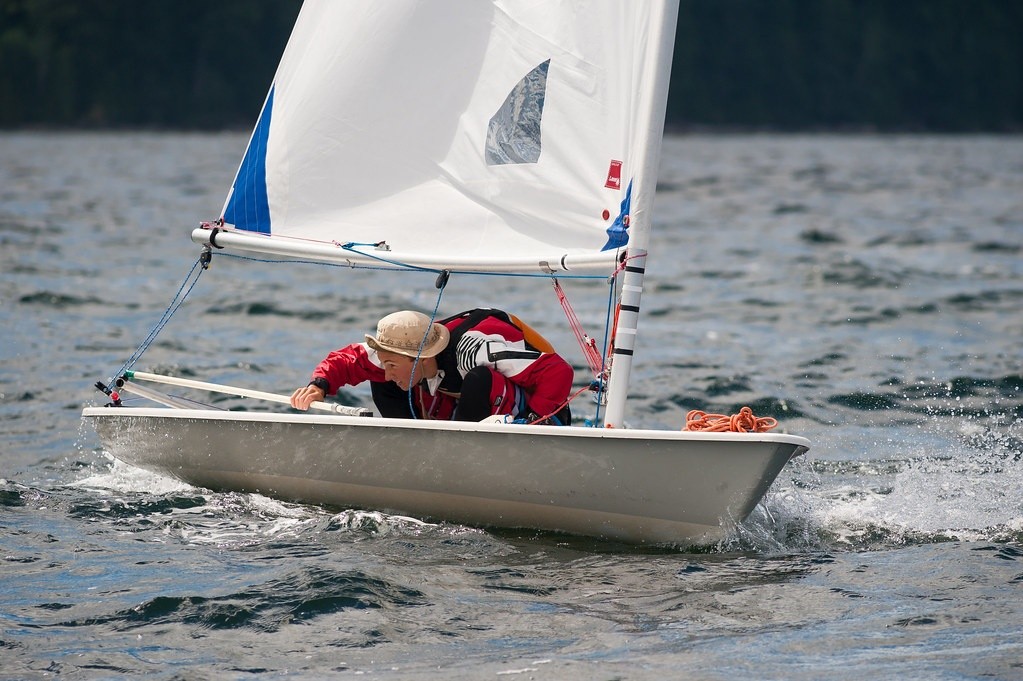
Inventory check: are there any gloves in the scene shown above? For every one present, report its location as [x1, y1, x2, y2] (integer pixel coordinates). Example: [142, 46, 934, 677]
[514, 405, 543, 425]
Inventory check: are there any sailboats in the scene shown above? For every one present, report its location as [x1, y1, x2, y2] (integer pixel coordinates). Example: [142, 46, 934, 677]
[84, 0, 813, 553]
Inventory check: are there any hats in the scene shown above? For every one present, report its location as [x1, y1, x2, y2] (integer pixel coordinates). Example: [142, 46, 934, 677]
[366, 311, 449, 357]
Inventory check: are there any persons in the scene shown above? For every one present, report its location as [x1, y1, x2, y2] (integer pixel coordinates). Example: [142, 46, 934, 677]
[290, 308, 574, 425]
